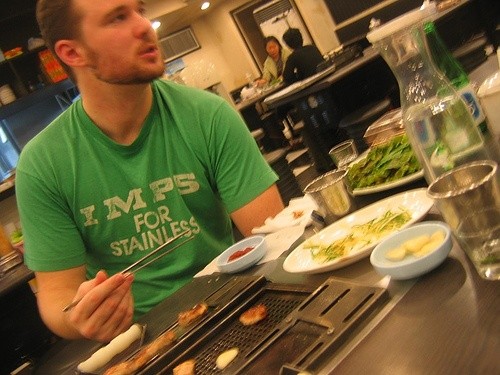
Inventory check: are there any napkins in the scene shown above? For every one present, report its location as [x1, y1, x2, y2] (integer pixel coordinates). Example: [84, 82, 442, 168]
[192, 194, 317, 279]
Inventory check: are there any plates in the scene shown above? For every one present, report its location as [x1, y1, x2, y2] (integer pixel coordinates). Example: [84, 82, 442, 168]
[347, 141, 485, 195]
[282, 187, 435, 275]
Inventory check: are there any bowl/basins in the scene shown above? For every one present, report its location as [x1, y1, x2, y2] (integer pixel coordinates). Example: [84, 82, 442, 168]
[214, 236, 267, 275]
[369, 223, 453, 280]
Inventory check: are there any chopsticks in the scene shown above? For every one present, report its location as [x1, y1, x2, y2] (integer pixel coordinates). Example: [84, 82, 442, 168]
[63, 228, 195, 312]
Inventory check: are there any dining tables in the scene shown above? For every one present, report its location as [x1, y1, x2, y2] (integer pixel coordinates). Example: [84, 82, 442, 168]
[0, 0, 500, 375]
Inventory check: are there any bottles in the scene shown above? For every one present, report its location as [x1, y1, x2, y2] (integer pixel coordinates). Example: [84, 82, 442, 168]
[412, 21, 489, 135]
[366, 11, 492, 183]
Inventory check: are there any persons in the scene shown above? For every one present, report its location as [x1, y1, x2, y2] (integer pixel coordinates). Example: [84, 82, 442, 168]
[253, 28, 325, 83]
[14, 0, 285, 343]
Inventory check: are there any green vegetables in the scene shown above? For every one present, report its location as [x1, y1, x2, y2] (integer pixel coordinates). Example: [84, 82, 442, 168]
[302, 207, 414, 264]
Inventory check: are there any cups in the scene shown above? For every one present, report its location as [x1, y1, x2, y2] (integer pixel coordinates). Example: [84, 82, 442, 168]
[302, 169, 358, 227]
[425, 160, 500, 281]
[328, 139, 357, 170]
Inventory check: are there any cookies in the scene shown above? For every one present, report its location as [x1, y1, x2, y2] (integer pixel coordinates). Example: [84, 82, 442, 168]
[386, 230, 445, 259]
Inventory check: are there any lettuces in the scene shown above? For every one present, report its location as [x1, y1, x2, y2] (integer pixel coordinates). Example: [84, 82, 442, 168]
[346, 135, 422, 190]
